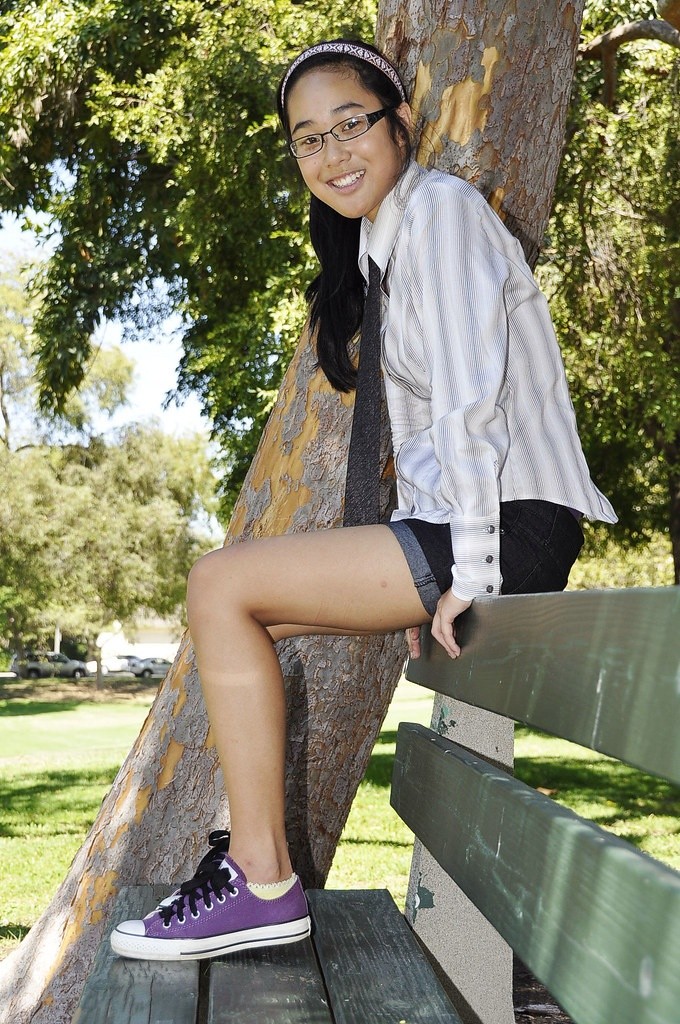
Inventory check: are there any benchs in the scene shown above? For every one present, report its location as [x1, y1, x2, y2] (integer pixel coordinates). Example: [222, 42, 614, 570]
[77, 584, 680, 1024]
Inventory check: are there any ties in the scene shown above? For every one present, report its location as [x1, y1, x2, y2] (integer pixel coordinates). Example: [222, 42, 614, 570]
[342, 255, 381, 527]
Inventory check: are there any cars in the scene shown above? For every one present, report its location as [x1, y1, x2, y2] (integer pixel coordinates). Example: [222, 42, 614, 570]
[101, 655, 141, 672]
[130, 658, 172, 678]
[11, 653, 90, 679]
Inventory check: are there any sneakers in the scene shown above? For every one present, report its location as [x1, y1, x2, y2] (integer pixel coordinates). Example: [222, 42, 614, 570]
[110, 854, 311, 961]
[144, 830, 231, 919]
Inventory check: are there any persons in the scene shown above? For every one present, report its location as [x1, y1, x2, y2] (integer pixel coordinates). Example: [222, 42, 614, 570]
[111, 36, 618, 961]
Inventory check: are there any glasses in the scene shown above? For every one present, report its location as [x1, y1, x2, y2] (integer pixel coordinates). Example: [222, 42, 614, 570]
[287, 108, 388, 159]
